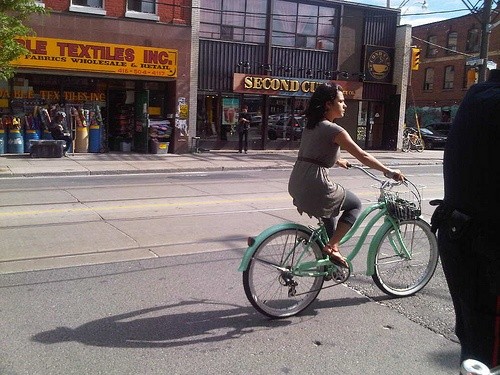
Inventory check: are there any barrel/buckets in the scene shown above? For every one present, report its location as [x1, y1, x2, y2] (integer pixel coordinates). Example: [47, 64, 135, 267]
[42, 130, 53, 140]
[89, 126, 100, 152]
[76, 127, 88, 152]
[0, 130, 7, 153]
[8, 130, 24, 152]
[25, 130, 40, 152]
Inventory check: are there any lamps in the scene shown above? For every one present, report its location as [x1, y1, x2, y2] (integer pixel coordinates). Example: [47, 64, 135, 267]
[235, 61, 349, 79]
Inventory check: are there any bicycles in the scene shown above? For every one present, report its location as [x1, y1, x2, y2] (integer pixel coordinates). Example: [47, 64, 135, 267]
[402, 129, 424, 152]
[238, 163, 439, 319]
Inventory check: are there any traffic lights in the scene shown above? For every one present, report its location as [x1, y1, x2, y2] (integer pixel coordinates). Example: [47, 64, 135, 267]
[412, 48, 421, 70]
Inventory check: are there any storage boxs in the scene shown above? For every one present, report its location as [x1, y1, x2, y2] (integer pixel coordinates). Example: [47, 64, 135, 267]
[119, 142, 132, 152]
[152, 142, 170, 154]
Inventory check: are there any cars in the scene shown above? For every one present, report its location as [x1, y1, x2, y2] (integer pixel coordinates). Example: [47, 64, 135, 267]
[249, 112, 306, 140]
[406, 127, 447, 150]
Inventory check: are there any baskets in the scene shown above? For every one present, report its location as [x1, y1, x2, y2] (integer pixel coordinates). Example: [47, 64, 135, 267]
[376, 181, 424, 220]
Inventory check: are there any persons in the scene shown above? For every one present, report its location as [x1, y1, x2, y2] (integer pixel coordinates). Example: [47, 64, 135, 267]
[288, 82, 408, 280]
[50, 111, 72, 157]
[237, 105, 250, 154]
[437, 69, 500, 375]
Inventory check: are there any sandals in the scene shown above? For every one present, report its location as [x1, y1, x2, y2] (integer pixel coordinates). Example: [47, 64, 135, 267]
[322, 245, 348, 268]
